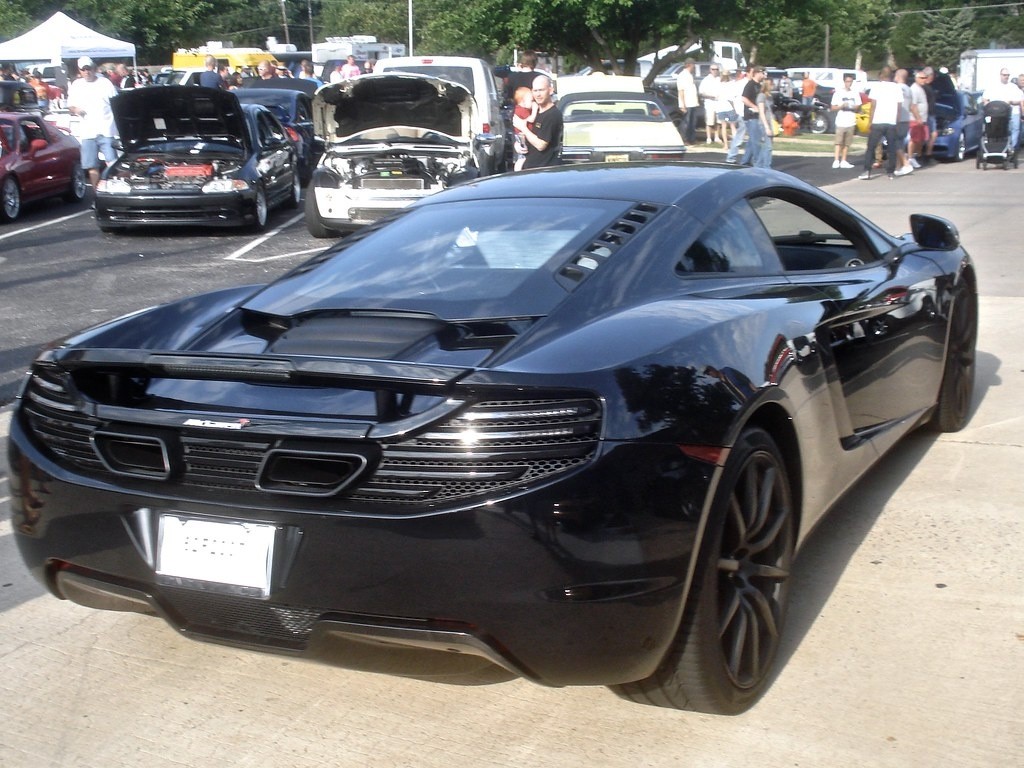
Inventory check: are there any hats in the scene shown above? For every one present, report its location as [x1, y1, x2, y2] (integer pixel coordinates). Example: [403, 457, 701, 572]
[753, 65, 765, 72]
[685, 57, 699, 64]
[78, 56, 92, 69]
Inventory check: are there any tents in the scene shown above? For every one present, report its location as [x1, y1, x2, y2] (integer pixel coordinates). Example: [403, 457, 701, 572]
[0, 11, 141, 85]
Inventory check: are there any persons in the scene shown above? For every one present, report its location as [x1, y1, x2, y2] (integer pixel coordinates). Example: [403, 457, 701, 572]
[830, 71, 863, 168]
[340, 54, 360, 80]
[778, 73, 796, 99]
[801, 70, 817, 118]
[501, 49, 550, 108]
[0, 61, 51, 118]
[199, 54, 295, 90]
[329, 64, 344, 83]
[67, 56, 155, 210]
[697, 65, 777, 170]
[298, 59, 323, 88]
[512, 74, 564, 169]
[981, 67, 1024, 154]
[513, 86, 539, 172]
[857, 66, 938, 180]
[676, 56, 700, 146]
[361, 59, 373, 75]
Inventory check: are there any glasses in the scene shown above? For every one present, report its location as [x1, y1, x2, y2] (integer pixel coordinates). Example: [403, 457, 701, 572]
[711, 69, 719, 71]
[1003, 74, 1010, 76]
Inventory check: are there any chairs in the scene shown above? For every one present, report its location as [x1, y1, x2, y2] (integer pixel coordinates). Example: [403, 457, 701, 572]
[7, 123, 35, 151]
[571, 110, 593, 116]
[623, 109, 646, 115]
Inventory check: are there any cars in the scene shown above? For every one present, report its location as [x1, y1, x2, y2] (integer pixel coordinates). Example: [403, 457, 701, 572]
[92, 55, 554, 237]
[556, 58, 868, 162]
[8, 161, 979, 716]
[882, 69, 984, 163]
[0, 81, 86, 224]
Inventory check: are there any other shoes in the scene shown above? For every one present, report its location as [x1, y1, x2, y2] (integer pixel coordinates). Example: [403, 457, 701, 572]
[714, 137, 722, 143]
[888, 173, 894, 179]
[691, 140, 701, 145]
[706, 137, 712, 144]
[908, 159, 921, 168]
[744, 163, 752, 166]
[684, 140, 689, 146]
[859, 170, 870, 180]
[895, 165, 914, 176]
[832, 160, 840, 169]
[840, 160, 854, 169]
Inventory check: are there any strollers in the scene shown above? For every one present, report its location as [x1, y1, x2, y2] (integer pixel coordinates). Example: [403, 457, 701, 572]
[976, 101, 1018, 171]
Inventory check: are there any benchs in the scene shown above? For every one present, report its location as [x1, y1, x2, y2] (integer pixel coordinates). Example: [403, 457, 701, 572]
[475, 231, 617, 270]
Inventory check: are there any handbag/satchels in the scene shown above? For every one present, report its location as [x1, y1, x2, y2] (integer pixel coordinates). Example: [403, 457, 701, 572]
[772, 119, 780, 137]
